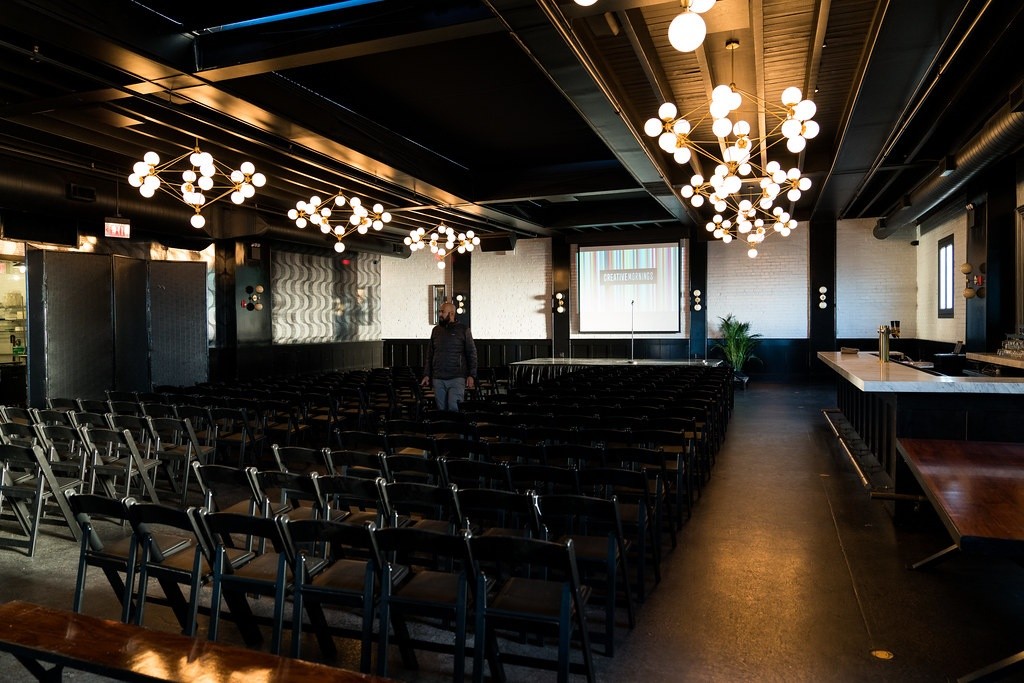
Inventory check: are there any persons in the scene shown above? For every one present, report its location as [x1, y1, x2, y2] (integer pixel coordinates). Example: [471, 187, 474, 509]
[420, 302, 478, 412]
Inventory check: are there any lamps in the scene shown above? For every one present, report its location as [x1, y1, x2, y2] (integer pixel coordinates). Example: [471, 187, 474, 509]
[246, 284, 264, 312]
[555, 293, 564, 314]
[643, 37, 820, 176]
[819, 286, 828, 309]
[455, 294, 464, 315]
[403, 221, 481, 269]
[680, 161, 812, 218]
[693, 289, 702, 312]
[287, 187, 392, 254]
[667, 0, 718, 54]
[961, 262, 976, 299]
[574, 0, 599, 6]
[705, 186, 798, 259]
[128, 135, 267, 230]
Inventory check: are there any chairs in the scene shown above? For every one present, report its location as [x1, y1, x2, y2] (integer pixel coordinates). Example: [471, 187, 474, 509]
[0, 363, 734, 683]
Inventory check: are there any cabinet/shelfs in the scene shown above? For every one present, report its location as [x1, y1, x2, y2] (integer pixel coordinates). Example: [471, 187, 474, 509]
[0, 303, 28, 339]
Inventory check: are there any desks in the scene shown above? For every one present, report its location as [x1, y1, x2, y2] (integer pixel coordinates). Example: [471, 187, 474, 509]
[508, 356, 724, 387]
[896, 436, 1024, 571]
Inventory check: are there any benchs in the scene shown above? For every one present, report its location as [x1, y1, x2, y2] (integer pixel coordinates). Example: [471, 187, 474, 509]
[0, 599, 399, 682]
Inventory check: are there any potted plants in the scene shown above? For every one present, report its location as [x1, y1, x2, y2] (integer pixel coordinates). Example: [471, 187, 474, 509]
[709, 312, 764, 382]
